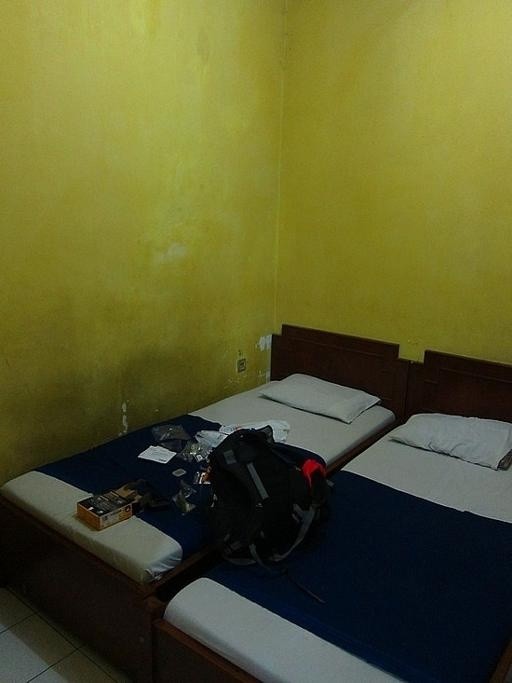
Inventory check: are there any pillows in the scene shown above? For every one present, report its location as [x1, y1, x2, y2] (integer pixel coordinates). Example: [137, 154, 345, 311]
[261, 371, 381, 425]
[390, 413, 511, 471]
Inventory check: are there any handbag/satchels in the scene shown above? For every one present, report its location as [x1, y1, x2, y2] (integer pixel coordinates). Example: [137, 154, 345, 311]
[208, 424, 329, 569]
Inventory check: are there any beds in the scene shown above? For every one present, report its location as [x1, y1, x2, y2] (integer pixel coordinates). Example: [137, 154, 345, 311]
[3, 376, 396, 682]
[158, 417, 511, 683]
